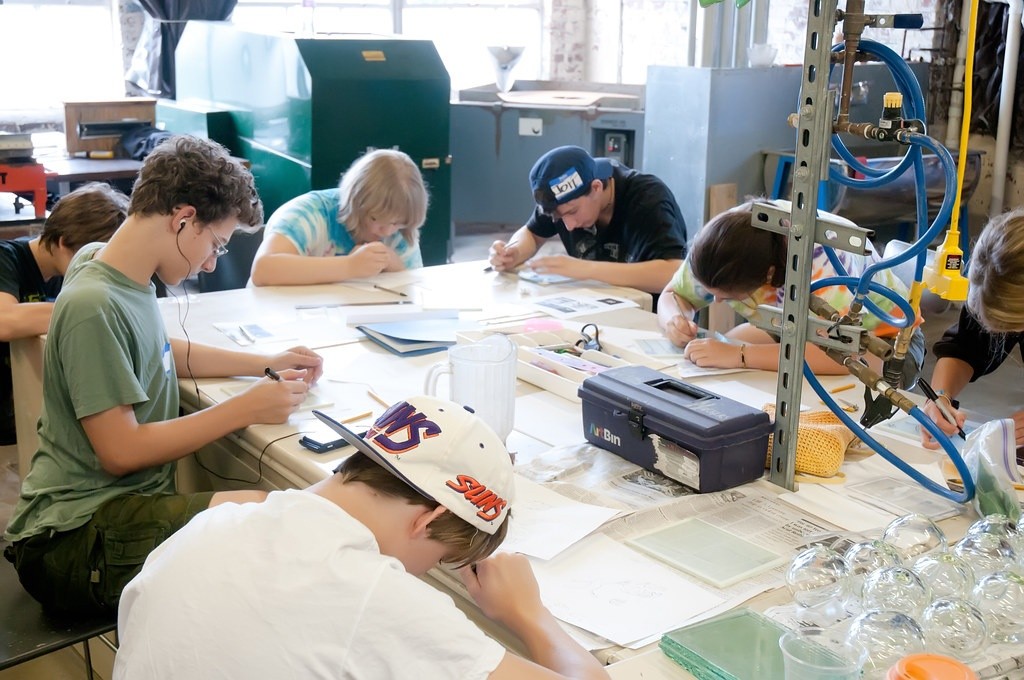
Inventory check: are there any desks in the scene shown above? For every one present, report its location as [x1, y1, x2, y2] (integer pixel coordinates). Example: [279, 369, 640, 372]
[9, 255, 1024, 680]
[30, 143, 250, 201]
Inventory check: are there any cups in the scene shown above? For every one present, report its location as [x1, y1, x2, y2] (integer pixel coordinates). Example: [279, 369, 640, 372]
[887, 653, 977, 680]
[779, 627, 868, 680]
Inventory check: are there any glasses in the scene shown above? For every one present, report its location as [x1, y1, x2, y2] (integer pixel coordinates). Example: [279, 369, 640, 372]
[173, 206, 229, 259]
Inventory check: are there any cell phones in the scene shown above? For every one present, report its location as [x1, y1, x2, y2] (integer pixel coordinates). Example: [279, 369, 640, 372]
[298, 426, 367, 453]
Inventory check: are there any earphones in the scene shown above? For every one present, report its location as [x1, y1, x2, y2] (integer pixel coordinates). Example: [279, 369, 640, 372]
[177, 219, 186, 234]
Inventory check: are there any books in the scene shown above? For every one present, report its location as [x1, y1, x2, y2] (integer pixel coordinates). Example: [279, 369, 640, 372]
[843, 474, 968, 523]
[626, 516, 791, 588]
[355, 318, 457, 358]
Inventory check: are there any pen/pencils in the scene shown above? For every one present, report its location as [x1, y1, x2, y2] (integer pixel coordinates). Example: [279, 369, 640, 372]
[368, 391, 389, 409]
[470, 562, 476, 573]
[671, 290, 696, 339]
[373, 285, 407, 296]
[340, 411, 373, 424]
[830, 384, 856, 394]
[264, 368, 285, 382]
[483, 266, 493, 272]
[504, 241, 519, 250]
[917, 377, 967, 441]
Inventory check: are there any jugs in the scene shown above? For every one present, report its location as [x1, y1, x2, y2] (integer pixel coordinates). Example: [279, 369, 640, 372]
[425, 332, 515, 446]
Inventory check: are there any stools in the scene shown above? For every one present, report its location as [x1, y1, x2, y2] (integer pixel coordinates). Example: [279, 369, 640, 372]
[0, 540, 121, 680]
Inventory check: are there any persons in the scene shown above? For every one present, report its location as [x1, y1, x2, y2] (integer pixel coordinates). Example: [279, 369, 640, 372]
[246, 148, 433, 289]
[0, 179, 167, 448]
[113, 398, 613, 680]
[1, 132, 323, 618]
[488, 144, 700, 326]
[660, 202, 926, 391]
[920, 204, 1024, 448]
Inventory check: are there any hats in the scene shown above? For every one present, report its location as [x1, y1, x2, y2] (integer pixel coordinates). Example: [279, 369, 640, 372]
[313, 395, 516, 537]
[530, 146, 613, 210]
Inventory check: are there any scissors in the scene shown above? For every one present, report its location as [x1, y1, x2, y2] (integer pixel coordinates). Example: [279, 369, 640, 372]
[575, 322, 621, 359]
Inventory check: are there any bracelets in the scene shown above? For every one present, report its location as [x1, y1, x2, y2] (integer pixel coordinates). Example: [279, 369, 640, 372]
[741, 344, 748, 369]
[924, 394, 953, 406]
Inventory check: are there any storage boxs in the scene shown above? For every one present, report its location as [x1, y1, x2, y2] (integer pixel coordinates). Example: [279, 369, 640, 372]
[64, 99, 157, 156]
[578, 362, 779, 494]
